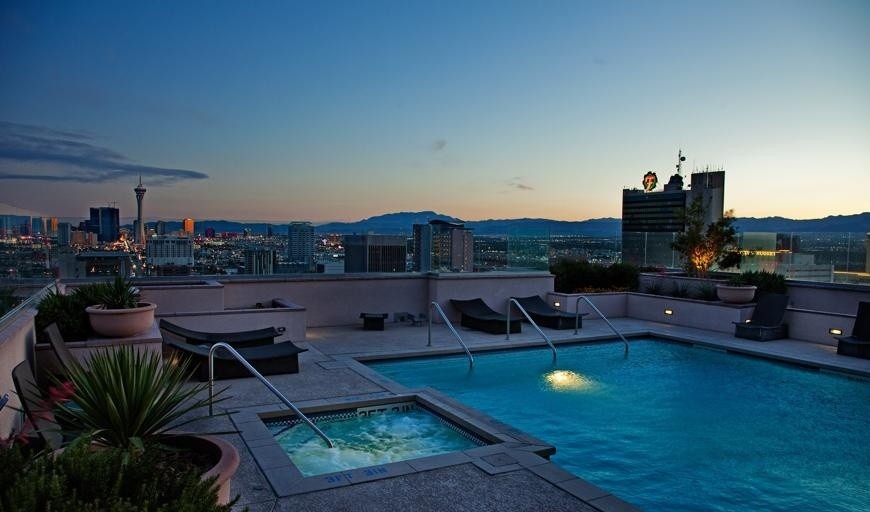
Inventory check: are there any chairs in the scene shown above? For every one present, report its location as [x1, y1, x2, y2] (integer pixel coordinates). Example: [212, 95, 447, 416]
[359, 313, 389, 330]
[159, 317, 310, 382]
[731, 291, 790, 342]
[448, 297, 524, 335]
[831, 300, 869, 361]
[510, 294, 590, 330]
[10, 321, 94, 449]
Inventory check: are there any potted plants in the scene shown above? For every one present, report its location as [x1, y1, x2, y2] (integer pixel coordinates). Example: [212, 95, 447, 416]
[716, 274, 758, 303]
[84, 271, 159, 339]
[0, 338, 242, 512]
[637, 186, 746, 300]
